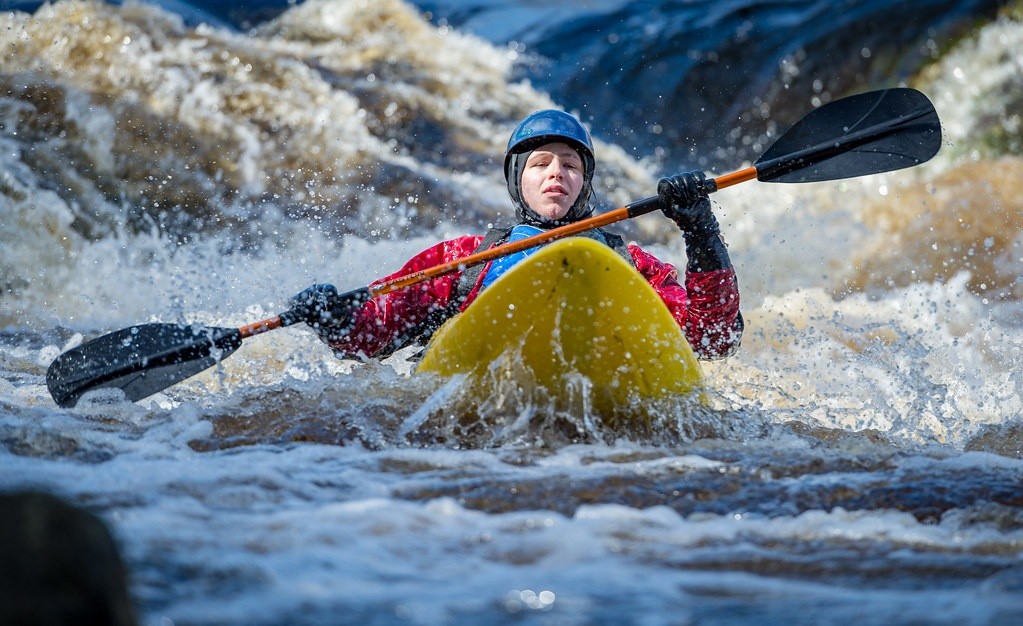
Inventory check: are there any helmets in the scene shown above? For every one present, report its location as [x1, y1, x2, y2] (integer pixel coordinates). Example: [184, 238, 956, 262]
[503, 109, 595, 183]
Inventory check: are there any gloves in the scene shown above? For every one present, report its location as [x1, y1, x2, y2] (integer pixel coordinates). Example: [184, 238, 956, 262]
[657, 171, 711, 229]
[288, 284, 347, 330]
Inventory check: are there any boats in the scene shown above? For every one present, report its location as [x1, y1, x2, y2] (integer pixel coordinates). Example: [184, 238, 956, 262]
[412, 236, 714, 415]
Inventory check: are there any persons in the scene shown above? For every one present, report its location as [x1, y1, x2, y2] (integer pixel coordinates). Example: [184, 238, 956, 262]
[290, 109, 744, 363]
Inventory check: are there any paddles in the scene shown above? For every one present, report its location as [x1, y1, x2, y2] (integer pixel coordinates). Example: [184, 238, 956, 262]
[42, 83, 948, 412]
